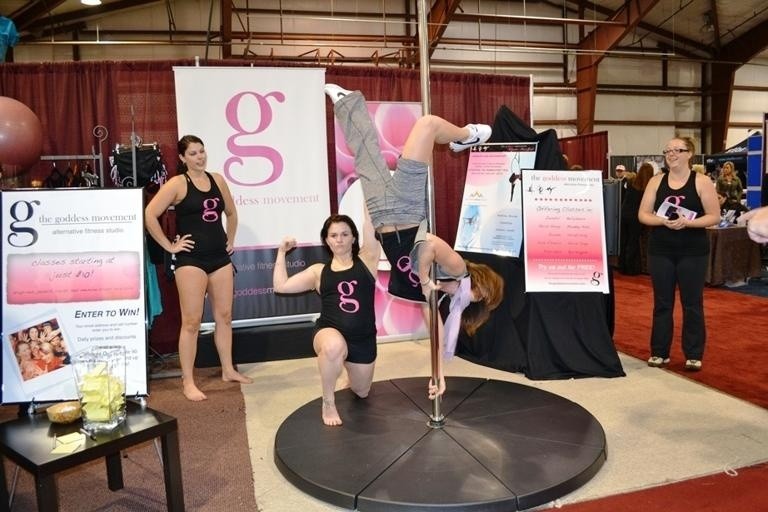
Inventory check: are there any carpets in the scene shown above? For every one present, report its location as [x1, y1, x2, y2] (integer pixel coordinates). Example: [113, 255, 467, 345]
[235, 337, 768, 511]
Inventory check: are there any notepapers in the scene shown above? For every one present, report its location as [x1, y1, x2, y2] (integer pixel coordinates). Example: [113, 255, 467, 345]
[81, 371, 124, 423]
[51, 444, 81, 455]
[56, 432, 82, 445]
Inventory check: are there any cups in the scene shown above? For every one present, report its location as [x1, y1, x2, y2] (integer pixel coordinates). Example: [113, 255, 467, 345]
[69, 344, 128, 435]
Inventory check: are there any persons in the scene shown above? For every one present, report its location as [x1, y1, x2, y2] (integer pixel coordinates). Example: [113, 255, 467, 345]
[322, 81, 502, 401]
[146, 135, 255, 401]
[637, 139, 720, 370]
[273, 197, 380, 426]
[715, 160, 742, 210]
[611, 163, 629, 205]
[620, 163, 655, 276]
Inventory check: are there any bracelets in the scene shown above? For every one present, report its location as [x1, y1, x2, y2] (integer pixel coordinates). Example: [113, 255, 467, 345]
[421, 276, 430, 287]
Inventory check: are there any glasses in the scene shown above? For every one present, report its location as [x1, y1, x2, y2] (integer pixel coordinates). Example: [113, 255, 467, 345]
[662, 148, 689, 154]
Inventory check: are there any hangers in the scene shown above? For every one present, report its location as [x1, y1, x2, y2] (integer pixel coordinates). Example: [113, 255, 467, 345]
[48, 159, 94, 186]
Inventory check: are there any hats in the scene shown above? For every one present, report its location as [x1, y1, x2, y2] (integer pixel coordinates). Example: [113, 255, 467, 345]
[615, 165, 626, 171]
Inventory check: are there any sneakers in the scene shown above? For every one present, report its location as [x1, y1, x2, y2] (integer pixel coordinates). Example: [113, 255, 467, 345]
[685, 359, 703, 371]
[646, 354, 671, 367]
[453, 123, 490, 151]
[321, 83, 351, 108]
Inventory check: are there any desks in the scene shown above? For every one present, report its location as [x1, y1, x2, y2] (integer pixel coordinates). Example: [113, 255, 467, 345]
[0, 399, 184, 512]
[706, 223, 761, 287]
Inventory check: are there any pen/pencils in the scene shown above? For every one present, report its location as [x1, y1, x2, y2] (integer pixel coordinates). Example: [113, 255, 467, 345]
[52, 432, 57, 449]
[80, 427, 97, 440]
